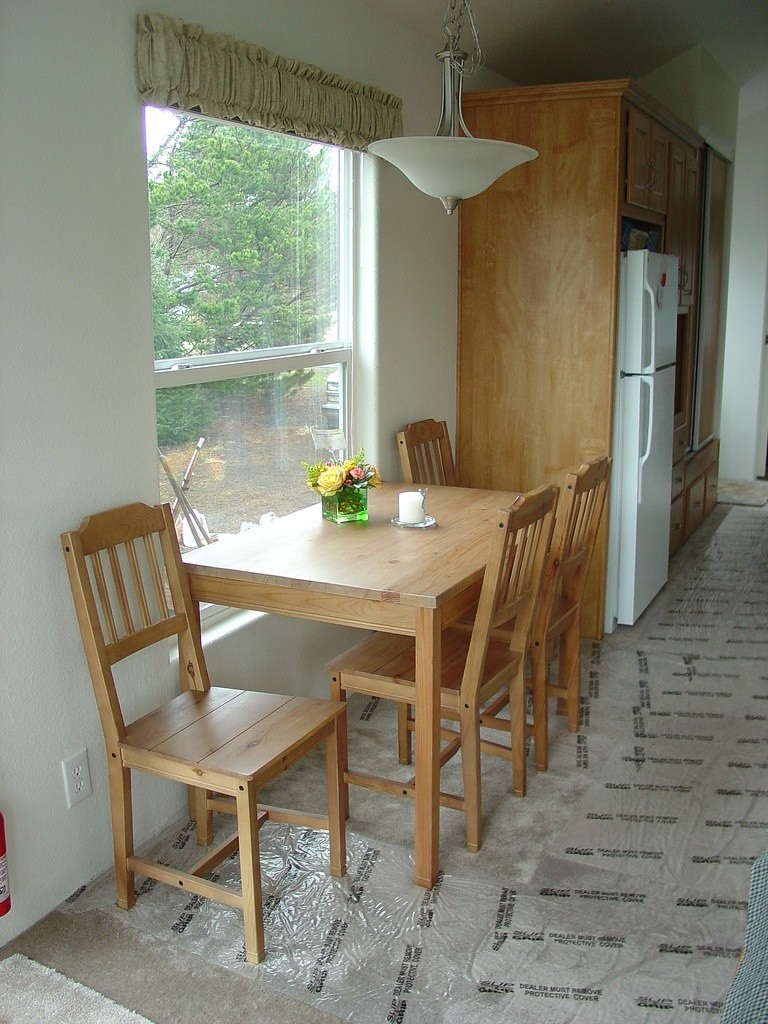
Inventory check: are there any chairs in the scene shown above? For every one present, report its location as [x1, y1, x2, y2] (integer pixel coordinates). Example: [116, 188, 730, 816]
[58, 502, 347, 963]
[394, 455, 615, 772]
[396, 417, 458, 487]
[322, 481, 565, 855]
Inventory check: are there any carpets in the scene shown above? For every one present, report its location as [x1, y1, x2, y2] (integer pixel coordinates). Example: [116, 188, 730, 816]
[717, 476, 768, 507]
[1, 953, 155, 1023]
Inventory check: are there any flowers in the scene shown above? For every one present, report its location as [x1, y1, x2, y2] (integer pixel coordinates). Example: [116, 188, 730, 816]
[301, 447, 382, 512]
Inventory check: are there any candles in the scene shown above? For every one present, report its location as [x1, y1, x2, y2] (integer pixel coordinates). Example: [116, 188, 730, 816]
[399, 491, 425, 523]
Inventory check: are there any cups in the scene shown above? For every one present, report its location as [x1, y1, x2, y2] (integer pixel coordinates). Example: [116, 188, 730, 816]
[397, 490, 426, 523]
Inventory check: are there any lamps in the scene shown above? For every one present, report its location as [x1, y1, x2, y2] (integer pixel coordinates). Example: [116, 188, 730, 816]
[366, 0, 538, 216]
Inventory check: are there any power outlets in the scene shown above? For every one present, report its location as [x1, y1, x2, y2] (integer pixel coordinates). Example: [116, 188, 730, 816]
[60, 748, 94, 812]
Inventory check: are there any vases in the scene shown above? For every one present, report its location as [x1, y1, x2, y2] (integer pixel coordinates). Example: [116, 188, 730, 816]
[319, 486, 368, 524]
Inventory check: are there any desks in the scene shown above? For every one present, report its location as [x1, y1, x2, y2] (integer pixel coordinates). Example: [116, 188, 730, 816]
[177, 481, 559, 891]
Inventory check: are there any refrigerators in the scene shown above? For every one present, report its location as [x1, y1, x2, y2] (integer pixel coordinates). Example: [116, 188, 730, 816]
[605, 249, 680, 636]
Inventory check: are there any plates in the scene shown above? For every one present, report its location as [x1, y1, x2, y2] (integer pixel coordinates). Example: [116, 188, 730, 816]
[391, 514, 435, 527]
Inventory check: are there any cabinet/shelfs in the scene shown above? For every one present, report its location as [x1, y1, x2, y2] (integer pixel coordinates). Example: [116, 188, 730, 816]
[454, 78, 729, 640]
